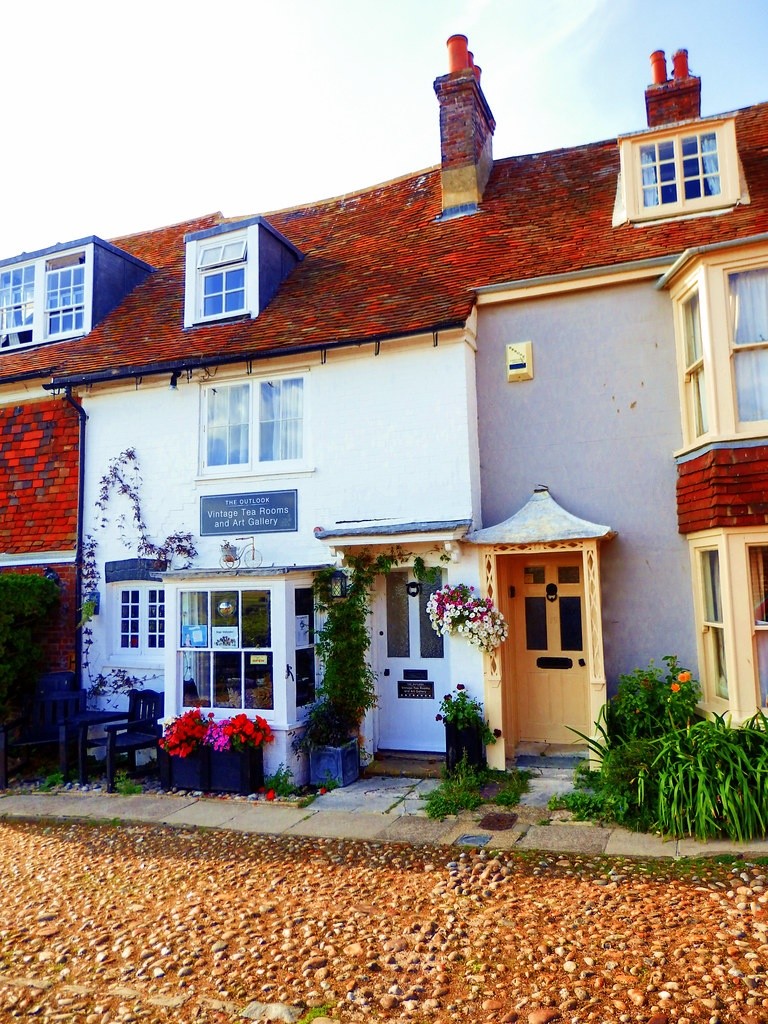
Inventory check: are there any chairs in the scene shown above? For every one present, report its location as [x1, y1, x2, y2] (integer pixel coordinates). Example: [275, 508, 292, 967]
[0, 688, 165, 794]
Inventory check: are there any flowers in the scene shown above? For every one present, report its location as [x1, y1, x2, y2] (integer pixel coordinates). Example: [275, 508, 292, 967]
[435, 683, 501, 746]
[157, 708, 274, 758]
[425, 584, 509, 654]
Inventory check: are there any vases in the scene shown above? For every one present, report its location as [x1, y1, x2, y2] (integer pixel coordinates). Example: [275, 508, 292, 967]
[171, 751, 210, 791]
[211, 749, 251, 793]
[446, 726, 482, 774]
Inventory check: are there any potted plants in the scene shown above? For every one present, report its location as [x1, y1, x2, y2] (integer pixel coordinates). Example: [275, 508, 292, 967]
[220, 539, 237, 558]
[287, 545, 451, 789]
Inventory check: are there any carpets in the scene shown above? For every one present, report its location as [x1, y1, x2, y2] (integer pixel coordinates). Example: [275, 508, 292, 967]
[515, 755, 584, 769]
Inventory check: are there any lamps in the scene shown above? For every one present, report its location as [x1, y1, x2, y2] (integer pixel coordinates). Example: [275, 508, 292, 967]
[217, 598, 237, 617]
[166, 369, 181, 392]
[331, 570, 349, 598]
[83, 584, 100, 615]
